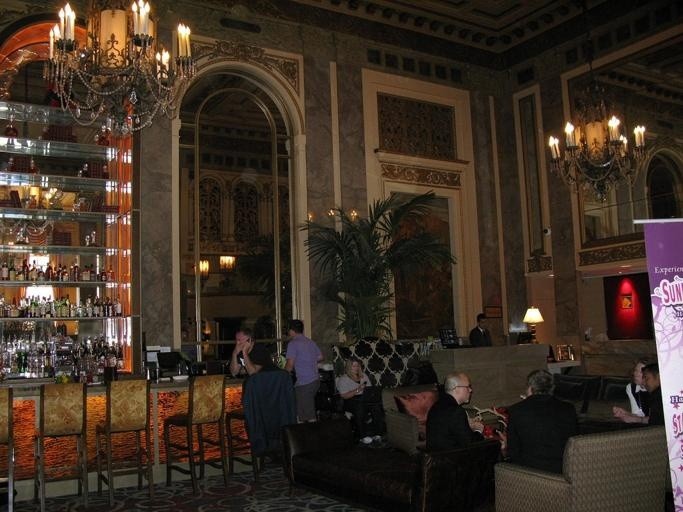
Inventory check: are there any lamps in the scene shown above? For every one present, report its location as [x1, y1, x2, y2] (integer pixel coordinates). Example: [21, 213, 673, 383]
[43, 1, 198, 147]
[193, 257, 210, 292]
[548, 35, 648, 201]
[218, 255, 235, 289]
[522, 305, 545, 343]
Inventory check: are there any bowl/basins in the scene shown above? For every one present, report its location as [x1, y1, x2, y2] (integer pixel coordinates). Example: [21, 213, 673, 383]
[173, 375, 189, 383]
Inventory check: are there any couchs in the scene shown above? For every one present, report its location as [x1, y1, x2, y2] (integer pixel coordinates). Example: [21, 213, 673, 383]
[281, 336, 678, 512]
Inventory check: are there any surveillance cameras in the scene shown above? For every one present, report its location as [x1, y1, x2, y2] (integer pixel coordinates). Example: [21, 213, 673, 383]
[543, 227, 551, 236]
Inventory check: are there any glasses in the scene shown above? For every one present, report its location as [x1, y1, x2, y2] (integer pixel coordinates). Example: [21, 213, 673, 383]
[456, 384, 471, 389]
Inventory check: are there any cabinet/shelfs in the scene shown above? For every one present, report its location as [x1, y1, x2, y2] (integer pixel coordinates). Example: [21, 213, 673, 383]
[0, 99, 135, 378]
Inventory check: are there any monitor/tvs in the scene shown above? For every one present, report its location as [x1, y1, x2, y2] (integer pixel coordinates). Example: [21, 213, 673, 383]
[517, 332, 533, 344]
[157, 352, 180, 368]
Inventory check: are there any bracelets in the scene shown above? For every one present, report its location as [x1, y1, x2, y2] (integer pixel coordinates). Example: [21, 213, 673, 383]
[640, 417, 644, 424]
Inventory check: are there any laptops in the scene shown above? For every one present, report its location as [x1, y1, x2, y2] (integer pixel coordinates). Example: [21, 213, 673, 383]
[439, 329, 472, 349]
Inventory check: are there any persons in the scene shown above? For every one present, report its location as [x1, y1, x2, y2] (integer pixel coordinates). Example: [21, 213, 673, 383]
[506, 368, 577, 473]
[229, 328, 277, 377]
[336, 360, 385, 444]
[469, 314, 490, 347]
[642, 363, 665, 425]
[285, 320, 323, 424]
[426, 373, 485, 453]
[612, 360, 652, 424]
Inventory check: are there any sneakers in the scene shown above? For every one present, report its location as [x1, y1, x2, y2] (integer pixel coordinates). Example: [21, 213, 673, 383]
[360, 437, 373, 444]
[373, 435, 381, 441]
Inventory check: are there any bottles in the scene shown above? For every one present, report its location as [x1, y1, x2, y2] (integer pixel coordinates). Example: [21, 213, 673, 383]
[2, 259, 115, 282]
[0, 322, 125, 379]
[10, 295, 122, 318]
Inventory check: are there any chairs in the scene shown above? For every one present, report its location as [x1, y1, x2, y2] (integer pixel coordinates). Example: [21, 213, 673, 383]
[226, 408, 263, 481]
[0, 386, 16, 512]
[32, 382, 89, 512]
[163, 374, 229, 494]
[94, 378, 153, 510]
